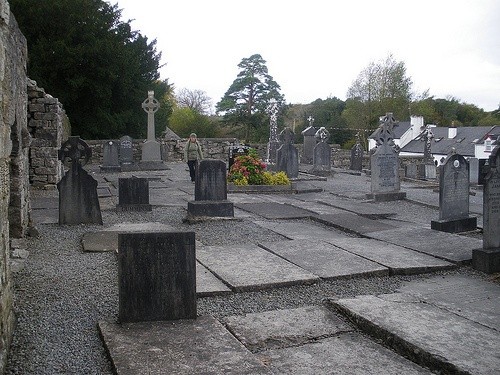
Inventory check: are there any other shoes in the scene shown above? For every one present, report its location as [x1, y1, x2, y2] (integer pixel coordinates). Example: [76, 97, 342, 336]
[193, 181, 195, 184]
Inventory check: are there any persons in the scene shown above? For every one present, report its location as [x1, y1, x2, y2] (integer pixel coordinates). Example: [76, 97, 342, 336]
[184, 133, 205, 183]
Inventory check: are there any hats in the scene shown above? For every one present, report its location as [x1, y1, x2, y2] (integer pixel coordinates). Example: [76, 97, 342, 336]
[190, 133, 196, 137]
